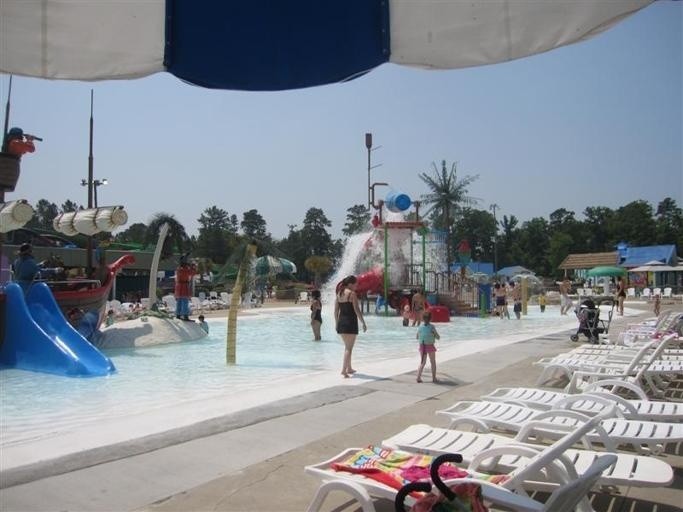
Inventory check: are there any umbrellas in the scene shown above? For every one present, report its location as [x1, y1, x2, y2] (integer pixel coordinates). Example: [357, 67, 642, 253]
[584, 265, 626, 295]
[626, 260, 682, 288]
[245, 254, 296, 289]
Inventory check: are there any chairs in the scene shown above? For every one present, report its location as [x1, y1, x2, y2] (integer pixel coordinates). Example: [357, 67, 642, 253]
[103, 289, 253, 322]
[574, 287, 674, 299]
[434, 401, 683, 457]
[379, 422, 675, 512]
[532, 308, 683, 400]
[302, 402, 619, 512]
[480, 386, 683, 426]
[297, 291, 309, 305]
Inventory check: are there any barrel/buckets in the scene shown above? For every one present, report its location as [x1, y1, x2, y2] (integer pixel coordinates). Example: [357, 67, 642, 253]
[384, 192, 412, 213]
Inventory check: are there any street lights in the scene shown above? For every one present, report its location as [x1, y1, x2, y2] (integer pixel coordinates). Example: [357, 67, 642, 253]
[78, 178, 108, 263]
[487, 202, 500, 281]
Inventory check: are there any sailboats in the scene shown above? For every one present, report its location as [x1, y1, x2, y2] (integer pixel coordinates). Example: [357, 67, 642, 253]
[0, 74, 135, 357]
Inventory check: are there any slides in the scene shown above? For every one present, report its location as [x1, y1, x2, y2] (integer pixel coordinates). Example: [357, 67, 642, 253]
[5, 281, 115, 377]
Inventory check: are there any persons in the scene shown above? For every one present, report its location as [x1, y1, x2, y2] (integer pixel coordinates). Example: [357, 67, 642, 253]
[332, 274, 368, 379]
[410, 288, 425, 328]
[308, 289, 323, 342]
[102, 309, 115, 329]
[197, 314, 209, 334]
[12, 243, 40, 281]
[401, 304, 410, 327]
[488, 274, 661, 320]
[173, 252, 196, 322]
[1, 127, 35, 158]
[413, 311, 440, 384]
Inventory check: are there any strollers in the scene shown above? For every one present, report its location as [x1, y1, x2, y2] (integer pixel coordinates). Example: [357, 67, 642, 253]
[393, 452, 491, 511]
[570, 297, 617, 343]
